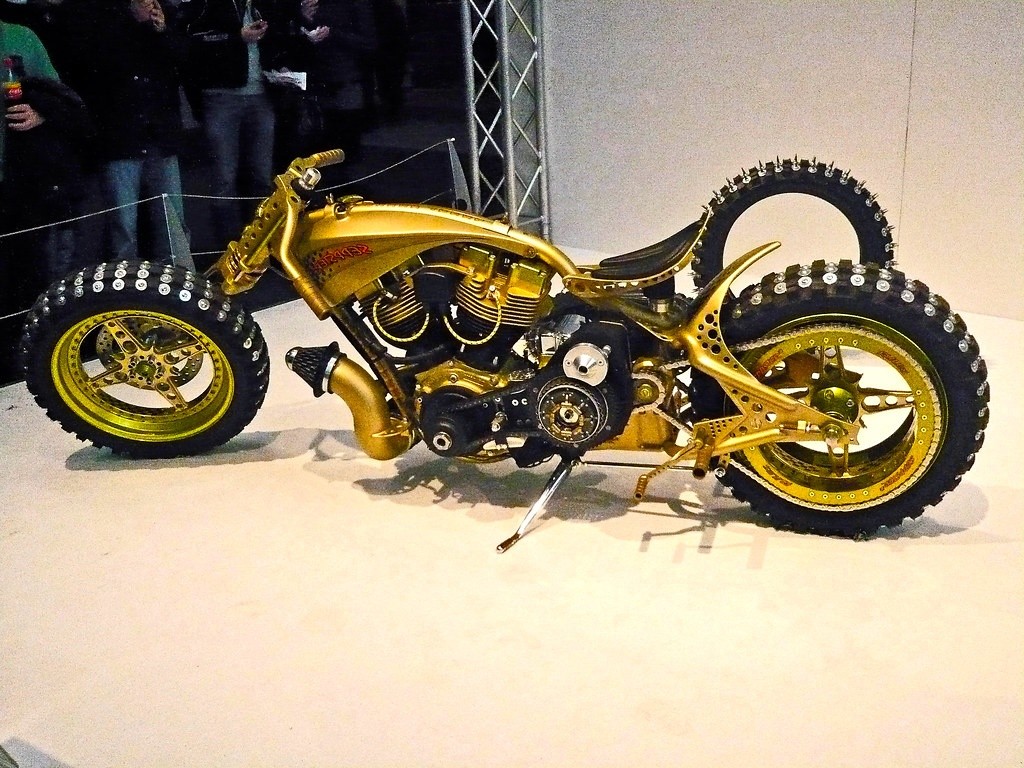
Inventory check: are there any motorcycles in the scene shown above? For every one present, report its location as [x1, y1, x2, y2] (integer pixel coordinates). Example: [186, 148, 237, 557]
[16, 144, 992, 539]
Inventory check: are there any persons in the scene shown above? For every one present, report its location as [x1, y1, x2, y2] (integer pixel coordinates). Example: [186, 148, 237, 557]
[0, 0, 505, 388]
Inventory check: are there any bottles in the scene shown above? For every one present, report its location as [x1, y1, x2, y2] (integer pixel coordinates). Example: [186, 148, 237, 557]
[1, 58, 25, 123]
